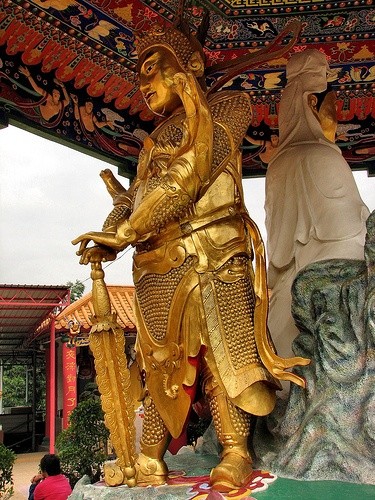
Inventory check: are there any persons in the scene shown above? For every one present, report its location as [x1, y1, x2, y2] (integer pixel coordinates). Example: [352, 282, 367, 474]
[27, 454, 72, 500]
[71, 18, 312, 494]
[266, 50, 371, 402]
